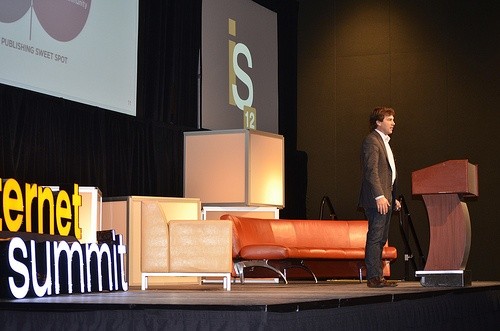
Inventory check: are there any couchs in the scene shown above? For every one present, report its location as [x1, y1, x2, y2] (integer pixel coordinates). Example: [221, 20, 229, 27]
[140, 201, 233, 292]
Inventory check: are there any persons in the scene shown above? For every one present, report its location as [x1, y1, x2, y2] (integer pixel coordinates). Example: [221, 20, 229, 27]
[357, 107, 401, 288]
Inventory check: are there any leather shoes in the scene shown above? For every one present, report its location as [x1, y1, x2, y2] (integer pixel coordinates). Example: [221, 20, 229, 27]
[367, 277, 398, 288]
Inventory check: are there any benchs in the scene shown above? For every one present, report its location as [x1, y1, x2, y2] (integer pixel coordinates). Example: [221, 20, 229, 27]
[220, 214, 397, 284]
